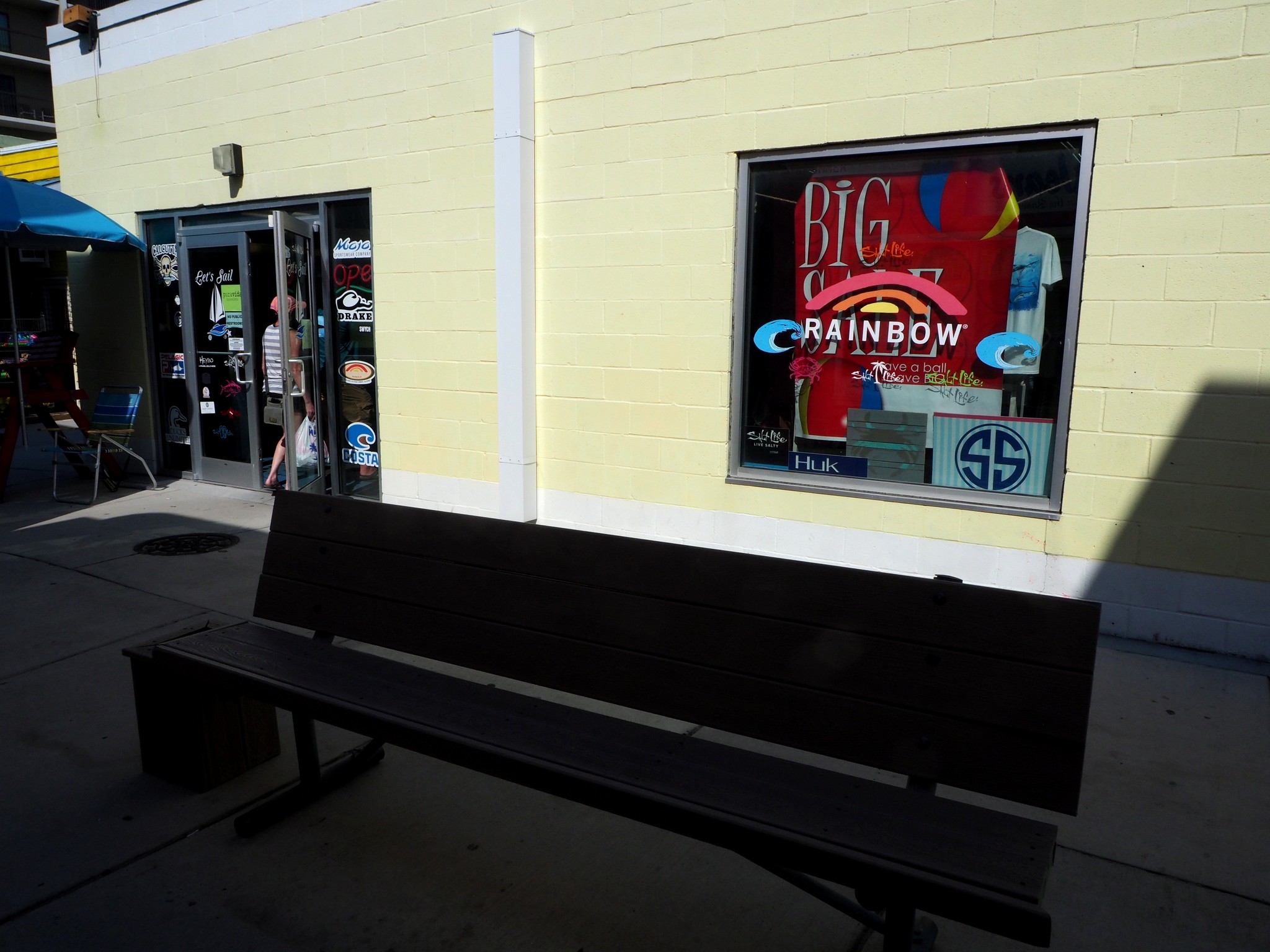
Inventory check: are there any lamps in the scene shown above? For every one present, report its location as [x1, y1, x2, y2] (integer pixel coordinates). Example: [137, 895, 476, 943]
[212, 143, 244, 177]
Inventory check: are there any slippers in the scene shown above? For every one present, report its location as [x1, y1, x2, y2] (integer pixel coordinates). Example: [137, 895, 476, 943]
[264, 482, 285, 490]
[356, 467, 379, 481]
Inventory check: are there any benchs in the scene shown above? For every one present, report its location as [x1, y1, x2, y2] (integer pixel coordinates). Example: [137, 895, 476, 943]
[156, 490, 1101, 952]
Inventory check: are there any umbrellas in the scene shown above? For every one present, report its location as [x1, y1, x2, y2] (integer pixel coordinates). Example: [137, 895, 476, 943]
[0, 173, 148, 448]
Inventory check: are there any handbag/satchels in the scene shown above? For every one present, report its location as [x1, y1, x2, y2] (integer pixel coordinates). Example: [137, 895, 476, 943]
[295, 415, 330, 469]
[263, 397, 284, 426]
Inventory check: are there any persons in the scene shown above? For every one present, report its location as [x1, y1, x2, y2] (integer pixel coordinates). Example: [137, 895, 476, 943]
[262, 294, 316, 490]
[319, 282, 382, 482]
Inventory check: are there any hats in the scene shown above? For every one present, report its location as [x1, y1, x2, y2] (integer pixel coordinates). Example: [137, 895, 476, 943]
[269, 294, 308, 315]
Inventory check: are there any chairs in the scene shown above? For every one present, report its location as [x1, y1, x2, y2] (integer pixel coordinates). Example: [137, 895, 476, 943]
[37, 386, 157, 505]
[0, 331, 126, 503]
